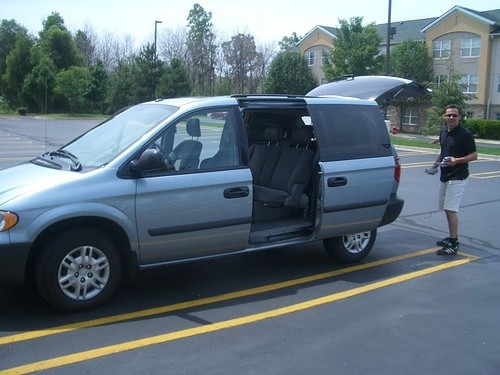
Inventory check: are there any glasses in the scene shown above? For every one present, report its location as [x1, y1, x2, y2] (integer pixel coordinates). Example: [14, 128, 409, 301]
[446, 114, 459, 117]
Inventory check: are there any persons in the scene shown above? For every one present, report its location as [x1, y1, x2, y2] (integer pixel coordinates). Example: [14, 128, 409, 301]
[433, 104, 478, 255]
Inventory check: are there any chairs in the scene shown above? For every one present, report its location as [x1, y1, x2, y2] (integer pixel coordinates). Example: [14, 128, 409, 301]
[200, 113, 240, 169]
[168, 118, 202, 171]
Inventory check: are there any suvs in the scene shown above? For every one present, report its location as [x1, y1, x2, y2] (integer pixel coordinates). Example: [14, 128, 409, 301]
[0, 75, 435, 317]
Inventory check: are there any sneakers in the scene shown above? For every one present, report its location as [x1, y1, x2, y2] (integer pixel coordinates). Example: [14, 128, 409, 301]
[436, 237, 459, 249]
[437, 244, 458, 255]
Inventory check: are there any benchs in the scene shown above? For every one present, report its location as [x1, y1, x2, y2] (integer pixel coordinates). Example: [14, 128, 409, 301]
[246, 126, 316, 221]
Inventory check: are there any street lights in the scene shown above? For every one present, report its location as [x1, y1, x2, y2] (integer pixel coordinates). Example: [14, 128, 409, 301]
[154, 21, 162, 61]
